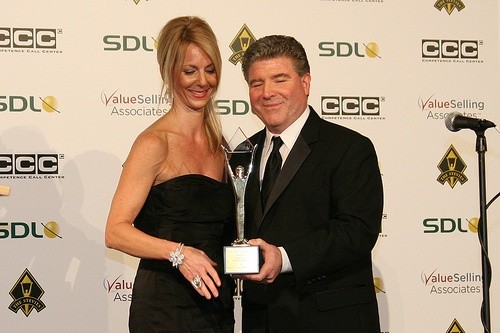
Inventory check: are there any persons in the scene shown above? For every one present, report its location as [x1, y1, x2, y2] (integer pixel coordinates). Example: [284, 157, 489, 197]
[105, 17, 238, 333]
[230, 34, 385, 333]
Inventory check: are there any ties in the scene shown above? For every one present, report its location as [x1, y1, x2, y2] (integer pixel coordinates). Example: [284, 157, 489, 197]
[261, 136, 284, 213]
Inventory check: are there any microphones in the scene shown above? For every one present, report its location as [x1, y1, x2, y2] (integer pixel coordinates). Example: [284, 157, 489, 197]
[445, 113, 496, 132]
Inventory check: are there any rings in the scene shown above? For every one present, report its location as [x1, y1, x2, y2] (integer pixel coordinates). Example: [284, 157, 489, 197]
[192, 275, 200, 286]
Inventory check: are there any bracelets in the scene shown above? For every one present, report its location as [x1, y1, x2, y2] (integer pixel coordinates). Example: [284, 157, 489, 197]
[170, 241, 184, 270]
